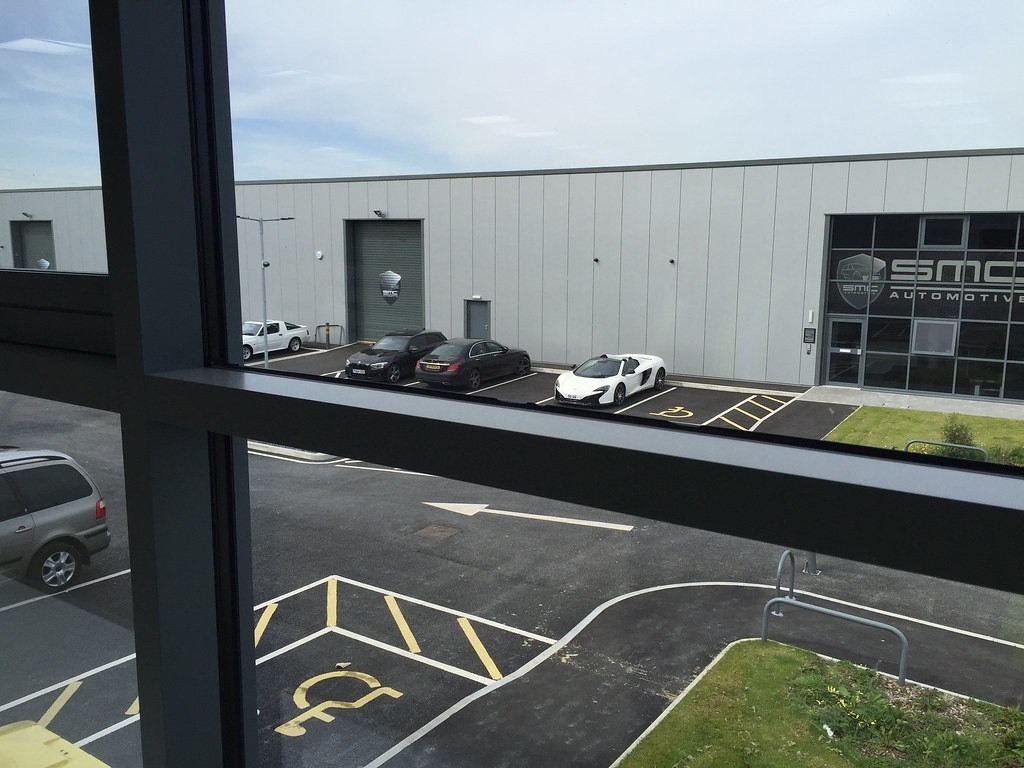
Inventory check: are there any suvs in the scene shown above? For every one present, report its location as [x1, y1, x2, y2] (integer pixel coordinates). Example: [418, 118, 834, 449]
[0, 446, 112, 595]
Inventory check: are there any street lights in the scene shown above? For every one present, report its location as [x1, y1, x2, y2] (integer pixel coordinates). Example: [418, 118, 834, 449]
[236, 214, 294, 369]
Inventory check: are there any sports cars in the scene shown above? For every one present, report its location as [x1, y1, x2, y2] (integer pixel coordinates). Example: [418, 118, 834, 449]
[555, 353, 668, 407]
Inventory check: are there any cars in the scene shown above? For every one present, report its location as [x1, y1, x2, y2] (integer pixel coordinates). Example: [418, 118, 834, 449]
[241, 320, 311, 361]
[345, 330, 447, 383]
[414, 339, 532, 391]
